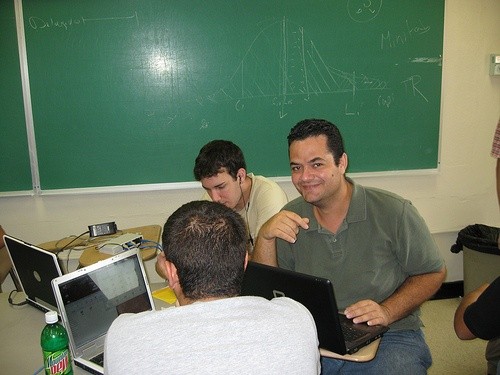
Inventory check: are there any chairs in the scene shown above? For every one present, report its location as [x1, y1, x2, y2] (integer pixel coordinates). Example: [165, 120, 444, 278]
[0, 225, 161, 292]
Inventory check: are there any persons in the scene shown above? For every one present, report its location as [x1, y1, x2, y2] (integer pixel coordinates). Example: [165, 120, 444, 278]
[491, 119, 500, 206]
[250, 119, 447, 375]
[155, 139, 288, 278]
[103, 200, 321, 375]
[454, 276, 500, 375]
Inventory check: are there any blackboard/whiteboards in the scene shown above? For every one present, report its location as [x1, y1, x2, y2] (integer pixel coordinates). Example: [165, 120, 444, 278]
[0, 0, 446, 197]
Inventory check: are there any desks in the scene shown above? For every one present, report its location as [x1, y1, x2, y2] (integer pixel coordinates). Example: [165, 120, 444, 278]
[0, 292, 94, 375]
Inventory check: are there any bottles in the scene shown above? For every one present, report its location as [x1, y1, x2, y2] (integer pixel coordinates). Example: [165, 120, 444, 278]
[40, 310, 74, 375]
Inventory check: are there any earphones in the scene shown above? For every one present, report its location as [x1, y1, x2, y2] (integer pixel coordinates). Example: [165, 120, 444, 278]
[239, 174, 241, 185]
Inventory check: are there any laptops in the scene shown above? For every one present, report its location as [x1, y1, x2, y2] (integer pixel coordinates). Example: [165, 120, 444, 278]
[4, 236, 65, 321]
[243, 260, 388, 356]
[50, 248, 155, 375]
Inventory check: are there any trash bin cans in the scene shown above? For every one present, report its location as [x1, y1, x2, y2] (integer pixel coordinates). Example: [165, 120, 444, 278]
[458, 224, 500, 298]
